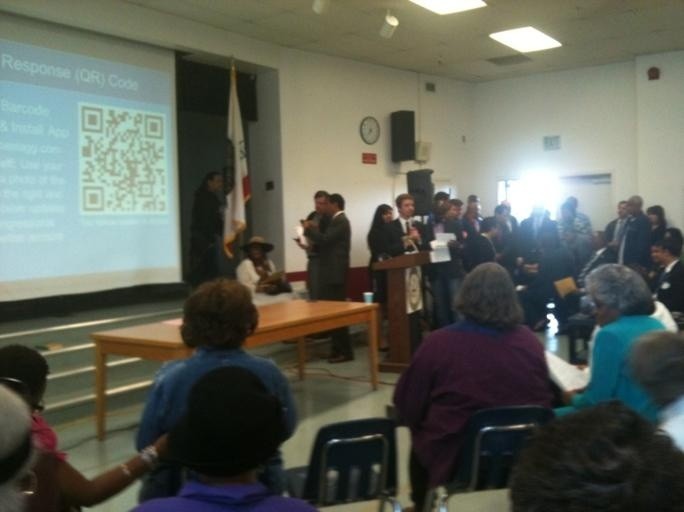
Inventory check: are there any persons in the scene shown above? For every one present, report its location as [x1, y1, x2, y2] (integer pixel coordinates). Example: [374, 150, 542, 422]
[523, 230, 570, 330]
[424, 191, 473, 328]
[602, 201, 628, 263]
[468, 194, 479, 201]
[631, 266, 679, 333]
[647, 206, 665, 234]
[0, 344, 166, 512]
[367, 205, 393, 353]
[555, 231, 615, 335]
[509, 401, 684, 511]
[236, 237, 298, 342]
[566, 197, 592, 235]
[620, 197, 651, 265]
[393, 262, 551, 512]
[630, 333, 684, 450]
[1, 381, 59, 512]
[562, 227, 581, 253]
[463, 204, 485, 235]
[129, 366, 318, 512]
[393, 194, 426, 256]
[553, 264, 669, 421]
[648, 242, 663, 295]
[653, 230, 684, 312]
[462, 217, 499, 273]
[502, 202, 517, 232]
[555, 204, 589, 241]
[301, 194, 354, 362]
[191, 171, 227, 279]
[294, 191, 334, 338]
[493, 204, 509, 224]
[131, 278, 298, 504]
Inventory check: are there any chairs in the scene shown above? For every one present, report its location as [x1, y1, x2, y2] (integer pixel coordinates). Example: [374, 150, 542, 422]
[281, 416, 416, 512]
[423, 404, 554, 512]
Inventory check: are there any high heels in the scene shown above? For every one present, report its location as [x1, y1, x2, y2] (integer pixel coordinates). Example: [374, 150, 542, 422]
[378, 346, 389, 353]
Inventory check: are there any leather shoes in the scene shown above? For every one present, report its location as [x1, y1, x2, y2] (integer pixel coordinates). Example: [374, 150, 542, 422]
[534, 317, 550, 331]
[328, 352, 350, 363]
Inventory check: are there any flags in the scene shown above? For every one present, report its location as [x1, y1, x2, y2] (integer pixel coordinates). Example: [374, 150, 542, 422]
[224, 55, 253, 259]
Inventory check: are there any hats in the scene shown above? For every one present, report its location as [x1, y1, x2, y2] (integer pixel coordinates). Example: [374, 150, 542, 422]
[239, 237, 274, 253]
[1, 379, 37, 496]
[435, 192, 450, 200]
[167, 366, 296, 471]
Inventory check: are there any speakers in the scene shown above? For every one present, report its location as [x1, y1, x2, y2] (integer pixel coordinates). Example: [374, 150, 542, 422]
[406, 169, 435, 216]
[390, 110, 416, 163]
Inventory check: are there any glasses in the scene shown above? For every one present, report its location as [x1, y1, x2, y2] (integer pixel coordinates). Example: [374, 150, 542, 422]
[590, 300, 617, 309]
[32, 401, 45, 416]
[618, 207, 627, 210]
[449, 209, 462, 212]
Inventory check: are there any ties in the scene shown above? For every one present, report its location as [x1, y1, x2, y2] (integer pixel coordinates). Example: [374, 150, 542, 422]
[578, 256, 597, 287]
[535, 218, 538, 238]
[406, 222, 410, 235]
[617, 222, 624, 241]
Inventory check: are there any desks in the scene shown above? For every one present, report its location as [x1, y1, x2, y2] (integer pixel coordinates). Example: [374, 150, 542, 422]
[89, 297, 379, 442]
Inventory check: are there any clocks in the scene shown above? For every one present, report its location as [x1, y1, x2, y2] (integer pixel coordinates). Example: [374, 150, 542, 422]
[360, 116, 380, 144]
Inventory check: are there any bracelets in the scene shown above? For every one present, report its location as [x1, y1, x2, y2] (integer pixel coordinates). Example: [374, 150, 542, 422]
[140, 445, 164, 470]
[120, 462, 135, 487]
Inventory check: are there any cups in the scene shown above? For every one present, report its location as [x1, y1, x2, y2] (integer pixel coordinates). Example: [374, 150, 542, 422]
[363, 292, 374, 304]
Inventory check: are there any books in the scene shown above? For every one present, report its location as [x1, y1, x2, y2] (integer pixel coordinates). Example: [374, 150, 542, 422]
[430, 232, 457, 263]
[554, 277, 577, 298]
[293, 225, 309, 247]
